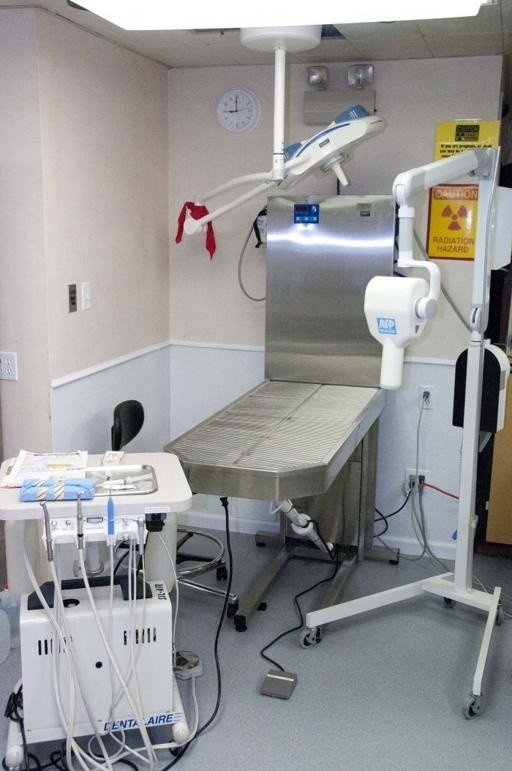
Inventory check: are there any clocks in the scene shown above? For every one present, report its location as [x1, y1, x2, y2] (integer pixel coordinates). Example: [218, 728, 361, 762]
[217, 85, 262, 135]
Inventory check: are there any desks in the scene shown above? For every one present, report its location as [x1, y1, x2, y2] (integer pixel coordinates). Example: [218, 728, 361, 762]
[165, 375, 385, 630]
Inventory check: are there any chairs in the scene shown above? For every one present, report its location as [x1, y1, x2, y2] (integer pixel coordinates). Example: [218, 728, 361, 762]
[106, 398, 240, 617]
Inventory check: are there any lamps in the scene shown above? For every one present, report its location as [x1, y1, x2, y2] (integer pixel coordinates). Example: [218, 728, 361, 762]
[301, 61, 378, 125]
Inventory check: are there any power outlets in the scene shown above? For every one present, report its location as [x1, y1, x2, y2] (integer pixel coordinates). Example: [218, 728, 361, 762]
[417, 384, 435, 409]
[406, 467, 430, 494]
[0, 351, 17, 380]
